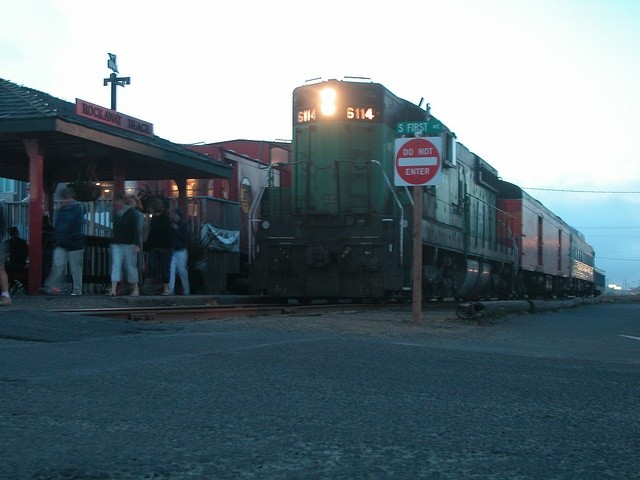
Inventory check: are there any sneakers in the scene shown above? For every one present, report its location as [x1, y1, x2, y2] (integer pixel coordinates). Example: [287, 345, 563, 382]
[0, 296, 12, 305]
[71, 292, 81, 296]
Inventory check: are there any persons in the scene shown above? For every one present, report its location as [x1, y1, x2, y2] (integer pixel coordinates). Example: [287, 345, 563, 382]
[161, 209, 190, 295]
[125, 195, 145, 295]
[0, 207, 12, 306]
[22, 183, 30, 202]
[4, 227, 30, 295]
[106, 190, 144, 296]
[38, 185, 84, 296]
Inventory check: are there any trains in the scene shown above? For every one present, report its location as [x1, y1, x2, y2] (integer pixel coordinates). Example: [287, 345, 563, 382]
[264, 80, 607, 298]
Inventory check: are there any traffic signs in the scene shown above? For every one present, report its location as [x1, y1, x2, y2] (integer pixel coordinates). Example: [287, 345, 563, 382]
[394, 138, 442, 186]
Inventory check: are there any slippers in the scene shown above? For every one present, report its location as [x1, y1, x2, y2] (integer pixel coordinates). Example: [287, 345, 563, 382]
[107, 292, 116, 296]
[132, 292, 140, 296]
